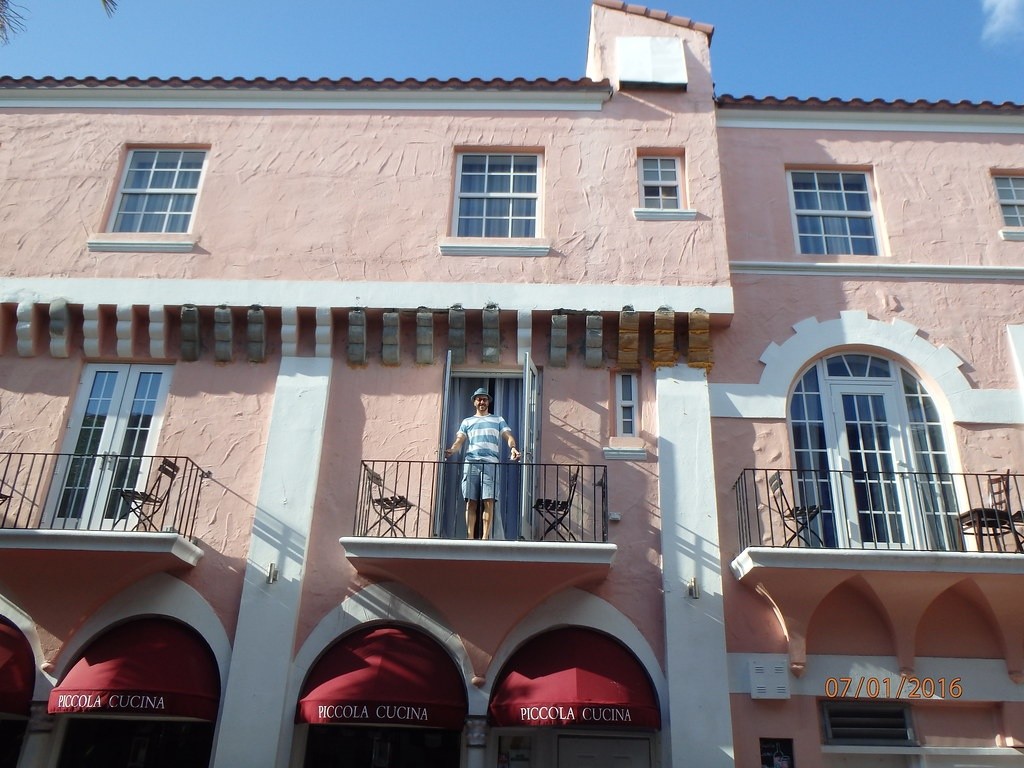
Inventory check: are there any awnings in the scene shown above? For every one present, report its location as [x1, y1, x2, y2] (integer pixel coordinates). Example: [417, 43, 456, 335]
[0, 614, 659, 728]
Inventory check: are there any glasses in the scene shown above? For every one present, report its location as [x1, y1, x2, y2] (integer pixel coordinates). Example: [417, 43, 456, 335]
[476, 398, 489, 402]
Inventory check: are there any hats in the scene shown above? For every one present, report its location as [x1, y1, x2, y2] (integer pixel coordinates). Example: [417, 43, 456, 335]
[471, 388, 492, 403]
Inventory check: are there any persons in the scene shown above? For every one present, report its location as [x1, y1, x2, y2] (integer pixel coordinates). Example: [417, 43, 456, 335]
[446, 387, 521, 540]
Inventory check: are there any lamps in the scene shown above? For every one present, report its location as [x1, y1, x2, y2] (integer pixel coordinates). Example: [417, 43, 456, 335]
[688, 577, 699, 599]
[267, 563, 278, 585]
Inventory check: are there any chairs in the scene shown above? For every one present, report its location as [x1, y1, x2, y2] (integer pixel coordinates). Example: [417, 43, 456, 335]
[956, 468, 1024, 554]
[532, 465, 580, 541]
[769, 472, 824, 548]
[111, 459, 180, 533]
[363, 465, 415, 538]
